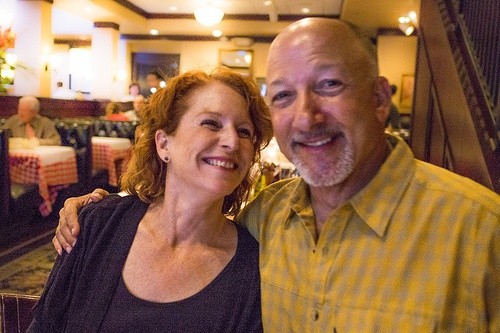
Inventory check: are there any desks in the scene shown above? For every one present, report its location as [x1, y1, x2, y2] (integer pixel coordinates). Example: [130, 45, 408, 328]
[7, 146, 79, 217]
[90, 134, 133, 188]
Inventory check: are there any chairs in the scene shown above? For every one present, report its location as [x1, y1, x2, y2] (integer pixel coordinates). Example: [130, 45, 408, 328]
[0, 116, 144, 246]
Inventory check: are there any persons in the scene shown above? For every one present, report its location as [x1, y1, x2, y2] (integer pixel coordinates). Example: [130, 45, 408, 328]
[51, 18, 500, 333]
[140, 72, 164, 98]
[384, 84, 402, 131]
[123, 82, 144, 101]
[0, 95, 62, 145]
[21, 68, 274, 333]
[126, 95, 145, 122]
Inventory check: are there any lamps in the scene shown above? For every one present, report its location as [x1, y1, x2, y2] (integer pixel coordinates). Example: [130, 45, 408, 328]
[399, 22, 415, 36]
